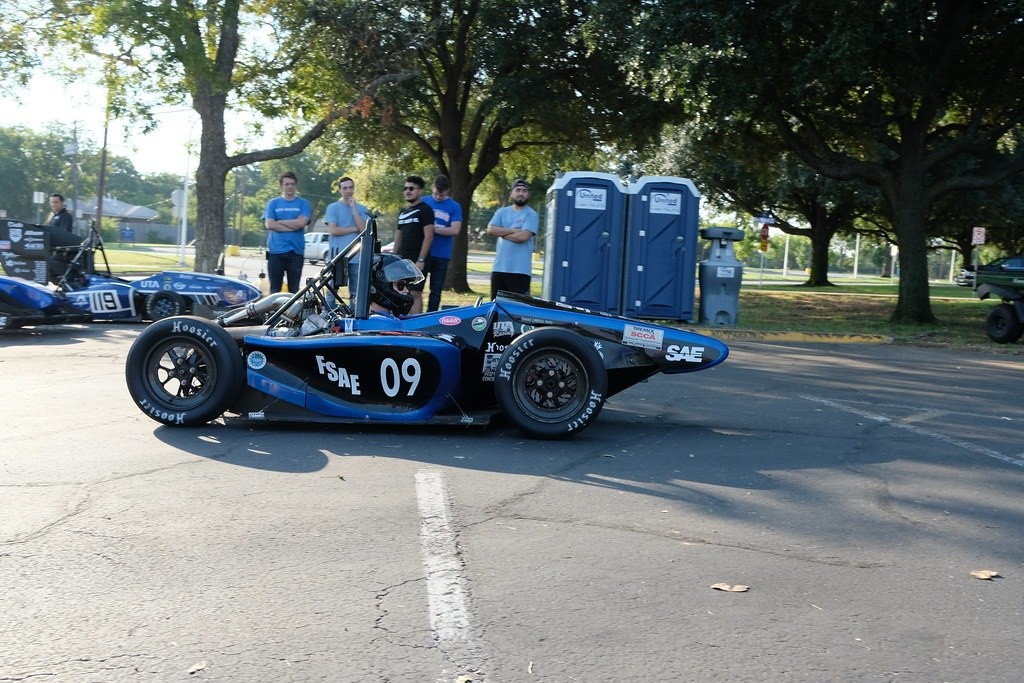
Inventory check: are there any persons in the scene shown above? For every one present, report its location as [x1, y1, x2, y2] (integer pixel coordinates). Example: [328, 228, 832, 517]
[421, 175, 462, 312]
[239, 271, 288, 292]
[488, 179, 538, 301]
[370, 253, 425, 316]
[393, 176, 435, 313]
[47, 194, 72, 233]
[325, 177, 368, 307]
[262, 172, 312, 293]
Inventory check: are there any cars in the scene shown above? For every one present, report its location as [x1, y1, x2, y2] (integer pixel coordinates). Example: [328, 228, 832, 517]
[124, 215, 729, 440]
[956, 256, 1024, 287]
[300, 233, 333, 265]
[977, 282, 1024, 344]
[0, 225, 266, 334]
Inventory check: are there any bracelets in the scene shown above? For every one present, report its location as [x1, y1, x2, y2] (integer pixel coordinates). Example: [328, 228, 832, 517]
[417, 257, 425, 262]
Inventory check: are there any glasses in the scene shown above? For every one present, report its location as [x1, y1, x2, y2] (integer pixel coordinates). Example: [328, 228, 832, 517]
[398, 281, 410, 291]
[404, 187, 421, 191]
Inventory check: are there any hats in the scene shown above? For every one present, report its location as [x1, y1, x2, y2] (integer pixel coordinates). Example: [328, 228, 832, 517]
[511, 179, 530, 191]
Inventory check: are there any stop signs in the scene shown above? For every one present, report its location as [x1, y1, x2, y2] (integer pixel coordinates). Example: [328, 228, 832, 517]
[761, 224, 769, 241]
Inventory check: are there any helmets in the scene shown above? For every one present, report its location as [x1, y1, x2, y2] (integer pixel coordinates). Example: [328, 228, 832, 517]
[370, 252, 425, 316]
